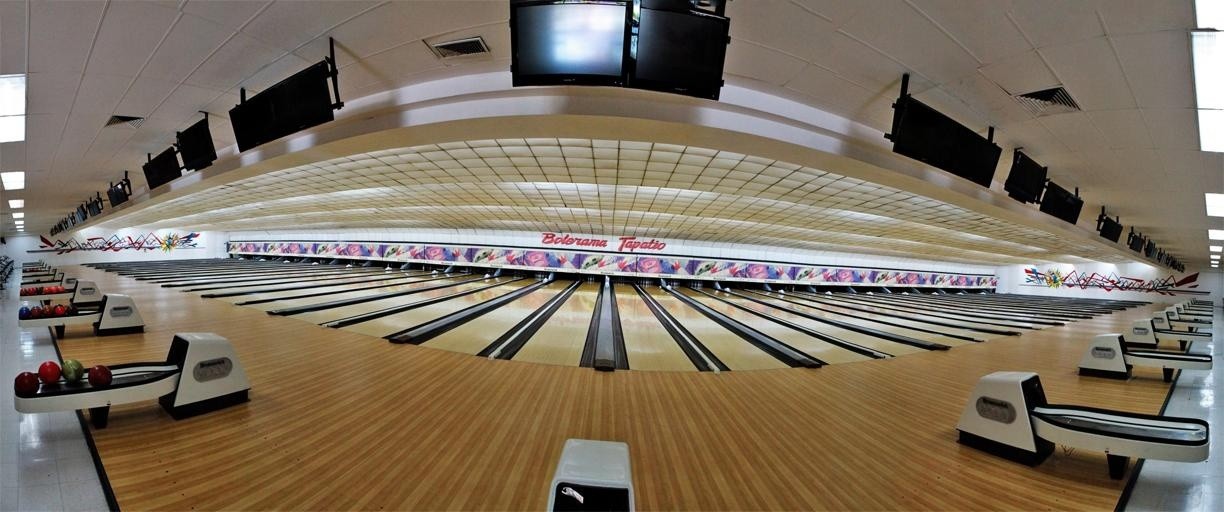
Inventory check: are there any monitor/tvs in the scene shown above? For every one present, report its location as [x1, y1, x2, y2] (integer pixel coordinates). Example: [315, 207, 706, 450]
[1129, 234, 1185, 274]
[175, 118, 218, 172]
[106, 181, 129, 208]
[1004, 150, 1044, 205]
[892, 96, 1003, 189]
[1099, 216, 1123, 244]
[142, 146, 183, 190]
[228, 59, 335, 153]
[509, 0, 633, 88]
[49, 199, 102, 237]
[627, 0, 731, 102]
[1038, 180, 1084, 226]
